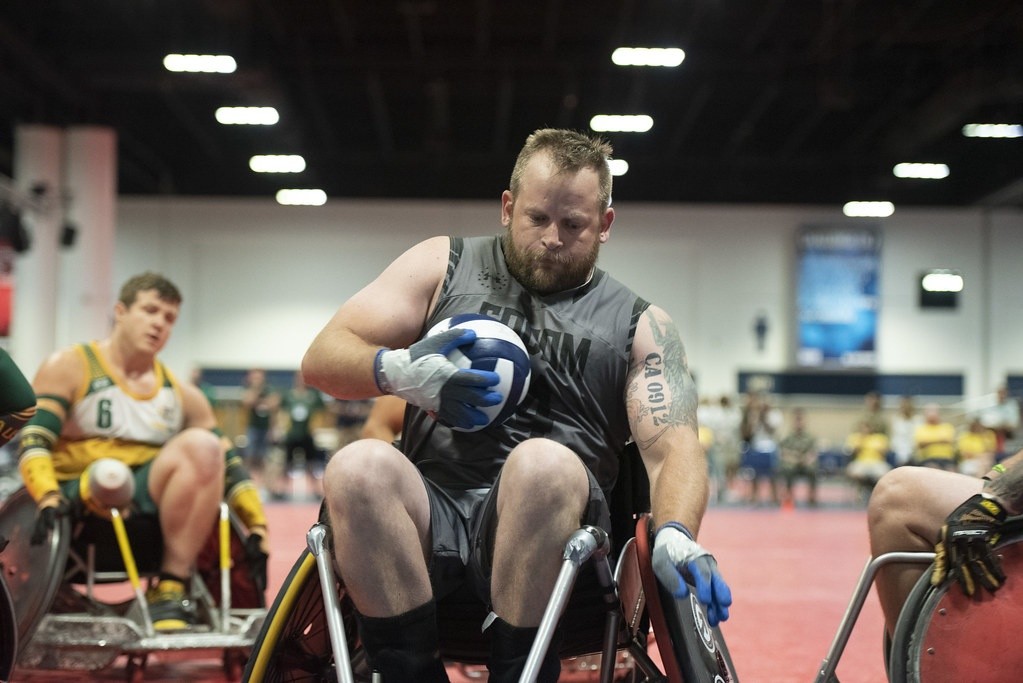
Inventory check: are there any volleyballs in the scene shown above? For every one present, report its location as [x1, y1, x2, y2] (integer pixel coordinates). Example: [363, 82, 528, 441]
[420, 313, 533, 431]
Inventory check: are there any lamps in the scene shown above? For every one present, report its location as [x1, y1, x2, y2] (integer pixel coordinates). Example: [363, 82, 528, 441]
[917, 264, 962, 307]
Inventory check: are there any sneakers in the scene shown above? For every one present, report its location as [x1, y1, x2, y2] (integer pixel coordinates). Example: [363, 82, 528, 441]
[147, 577, 188, 631]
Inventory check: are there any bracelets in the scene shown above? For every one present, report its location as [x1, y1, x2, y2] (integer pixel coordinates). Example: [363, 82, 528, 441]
[656, 521, 693, 539]
[992, 464, 1006, 473]
[374, 348, 390, 395]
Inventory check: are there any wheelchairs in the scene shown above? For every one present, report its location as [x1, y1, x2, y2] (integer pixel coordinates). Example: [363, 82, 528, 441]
[814, 516, 1022, 683]
[0, 481, 271, 683]
[241, 439, 740, 683]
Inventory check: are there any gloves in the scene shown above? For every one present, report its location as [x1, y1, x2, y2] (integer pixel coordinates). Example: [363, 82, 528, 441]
[245, 525, 270, 594]
[372, 328, 503, 429]
[930, 491, 1007, 597]
[28, 492, 79, 546]
[651, 521, 732, 625]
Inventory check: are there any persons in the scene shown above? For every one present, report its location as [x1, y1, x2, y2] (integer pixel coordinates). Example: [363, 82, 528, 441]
[301, 127, 731, 683]
[180, 364, 408, 503]
[686, 384, 1023, 508]
[865, 448, 1023, 645]
[0, 197, 36, 447]
[14, 270, 270, 632]
